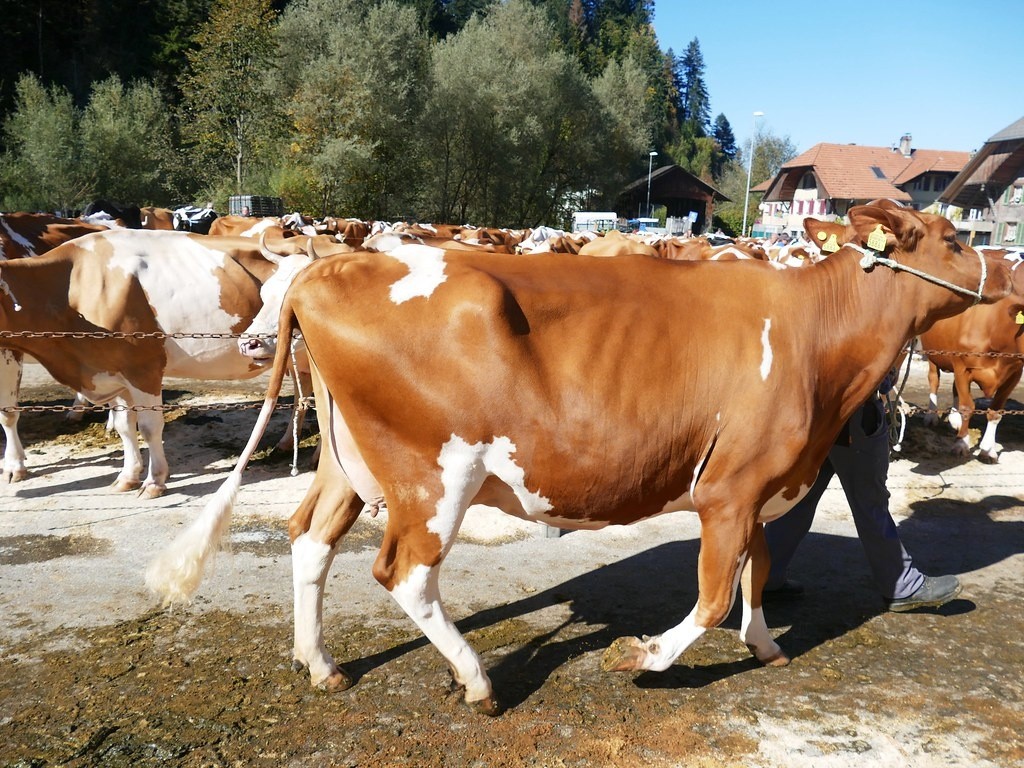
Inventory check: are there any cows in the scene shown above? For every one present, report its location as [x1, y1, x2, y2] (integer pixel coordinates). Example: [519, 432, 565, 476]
[920, 244, 1024, 465]
[144, 196, 1014, 713]
[1, 203, 833, 500]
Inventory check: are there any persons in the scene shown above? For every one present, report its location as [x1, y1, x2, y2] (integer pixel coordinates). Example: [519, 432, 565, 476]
[763, 383, 962, 613]
[760, 233, 779, 248]
[207, 203, 221, 218]
[777, 233, 791, 247]
[715, 228, 725, 236]
[241, 206, 251, 217]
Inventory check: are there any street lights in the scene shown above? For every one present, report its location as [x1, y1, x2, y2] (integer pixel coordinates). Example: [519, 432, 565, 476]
[645, 152, 659, 218]
[741, 111, 764, 236]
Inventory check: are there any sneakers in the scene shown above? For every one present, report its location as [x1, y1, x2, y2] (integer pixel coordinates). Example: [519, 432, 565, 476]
[762, 578, 803, 599]
[882, 573, 962, 612]
